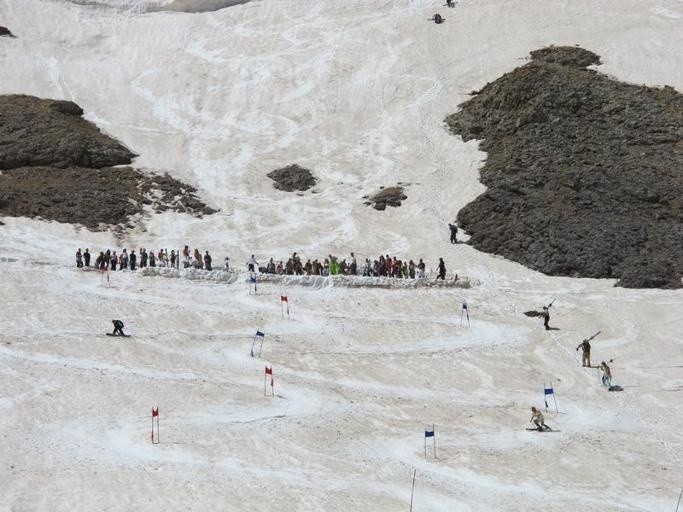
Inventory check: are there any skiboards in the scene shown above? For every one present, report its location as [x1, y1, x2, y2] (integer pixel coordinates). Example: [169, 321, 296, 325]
[527, 427, 561, 432]
[107, 333, 132, 337]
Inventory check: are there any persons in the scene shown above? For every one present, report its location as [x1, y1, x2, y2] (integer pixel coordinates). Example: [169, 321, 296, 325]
[222, 258, 229, 273]
[528, 406, 550, 432]
[245, 254, 258, 272]
[447, 222, 458, 245]
[93, 245, 213, 275]
[82, 247, 90, 266]
[575, 339, 592, 367]
[74, 248, 83, 268]
[598, 361, 612, 386]
[258, 251, 425, 279]
[110, 318, 124, 335]
[541, 306, 550, 330]
[436, 257, 446, 279]
